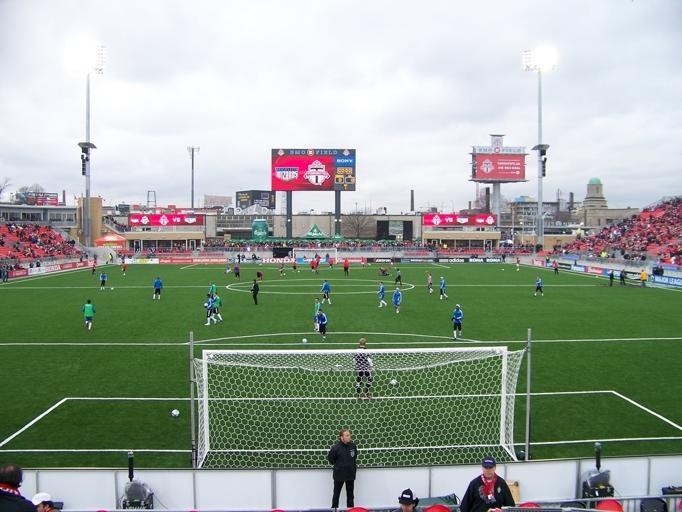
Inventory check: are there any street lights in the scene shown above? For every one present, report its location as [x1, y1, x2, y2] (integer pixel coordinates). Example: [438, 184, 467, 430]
[79, 40, 106, 245]
[187, 145, 201, 209]
[523, 49, 557, 249]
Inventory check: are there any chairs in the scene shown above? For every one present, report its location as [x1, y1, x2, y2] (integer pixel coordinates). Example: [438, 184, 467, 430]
[562, 195, 680, 267]
[437, 246, 550, 256]
[425, 504, 450, 510]
[117, 250, 223, 257]
[640, 498, 668, 510]
[518, 502, 541, 508]
[560, 501, 586, 509]
[349, 506, 368, 510]
[597, 500, 623, 510]
[0, 225, 82, 261]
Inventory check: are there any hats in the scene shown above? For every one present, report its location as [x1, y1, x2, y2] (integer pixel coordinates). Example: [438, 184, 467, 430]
[31, 492, 51, 506]
[398, 488, 419, 508]
[482, 457, 496, 468]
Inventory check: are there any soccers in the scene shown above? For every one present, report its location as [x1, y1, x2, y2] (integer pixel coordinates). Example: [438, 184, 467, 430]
[391, 379, 397, 385]
[204, 303, 208, 307]
[302, 339, 306, 343]
[171, 409, 179, 418]
[110, 288, 113, 290]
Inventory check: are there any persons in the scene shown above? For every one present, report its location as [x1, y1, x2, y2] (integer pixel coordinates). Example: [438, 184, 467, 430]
[391, 488, 420, 512]
[352, 337, 374, 400]
[456, 456, 516, 512]
[316, 309, 328, 343]
[313, 298, 322, 332]
[327, 429, 358, 512]
[204, 293, 217, 326]
[30, 492, 62, 512]
[0, 464, 52, 512]
[82, 299, 96, 331]
[212, 292, 223, 322]
[376, 282, 388, 308]
[392, 287, 402, 314]
[452, 303, 464, 341]
